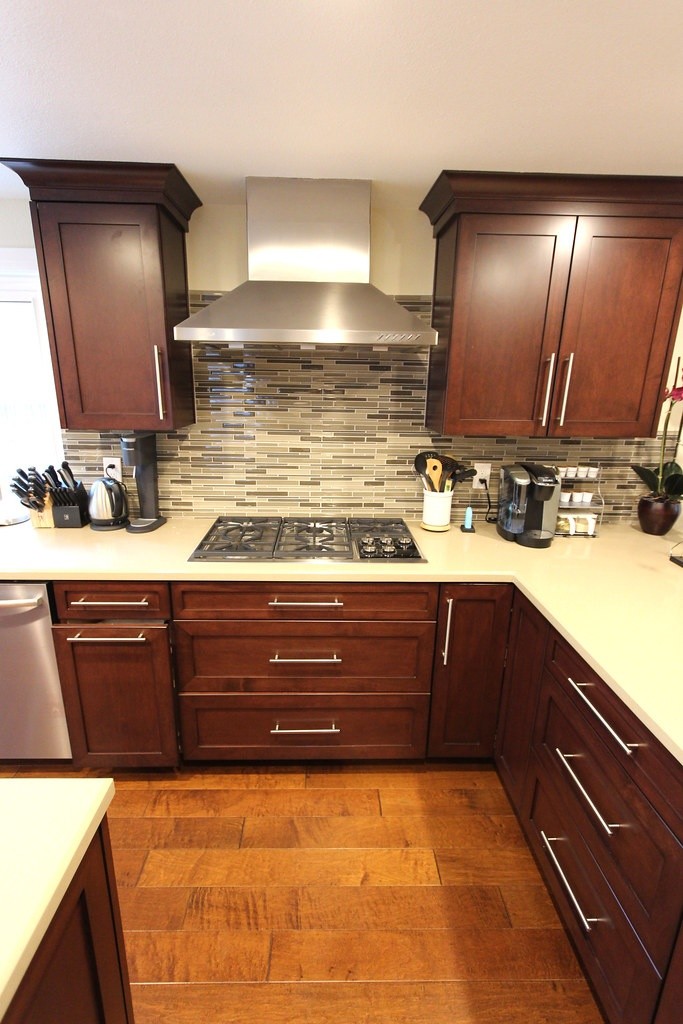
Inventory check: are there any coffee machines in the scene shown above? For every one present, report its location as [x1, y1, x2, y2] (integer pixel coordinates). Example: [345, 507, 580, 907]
[493, 461, 561, 548]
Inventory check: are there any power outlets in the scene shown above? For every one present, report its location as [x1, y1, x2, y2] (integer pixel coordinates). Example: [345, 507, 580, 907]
[472, 463, 491, 489]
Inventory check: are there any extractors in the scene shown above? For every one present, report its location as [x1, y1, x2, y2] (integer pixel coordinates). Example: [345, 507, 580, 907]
[171, 171, 437, 357]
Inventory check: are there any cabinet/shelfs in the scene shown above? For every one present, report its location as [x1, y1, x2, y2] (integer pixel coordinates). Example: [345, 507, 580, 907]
[0, 777, 136, 1024]
[0, 156, 204, 430]
[418, 171, 683, 441]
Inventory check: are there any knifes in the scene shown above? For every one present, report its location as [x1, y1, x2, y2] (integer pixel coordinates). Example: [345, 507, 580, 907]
[11, 464, 83, 512]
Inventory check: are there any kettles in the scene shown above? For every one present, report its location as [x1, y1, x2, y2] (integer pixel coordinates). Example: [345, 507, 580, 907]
[90, 477, 130, 532]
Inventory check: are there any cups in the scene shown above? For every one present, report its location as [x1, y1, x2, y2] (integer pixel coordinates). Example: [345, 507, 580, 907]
[573, 492, 583, 502]
[583, 492, 594, 502]
[560, 491, 572, 502]
[419, 489, 455, 532]
[557, 465, 567, 477]
[577, 466, 589, 477]
[566, 466, 578, 477]
[587, 467, 599, 477]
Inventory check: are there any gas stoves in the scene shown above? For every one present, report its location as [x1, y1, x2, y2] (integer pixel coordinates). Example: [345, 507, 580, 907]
[186, 506, 425, 567]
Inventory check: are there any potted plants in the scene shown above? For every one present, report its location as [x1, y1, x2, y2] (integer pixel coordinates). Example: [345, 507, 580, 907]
[632, 386, 683, 536]
